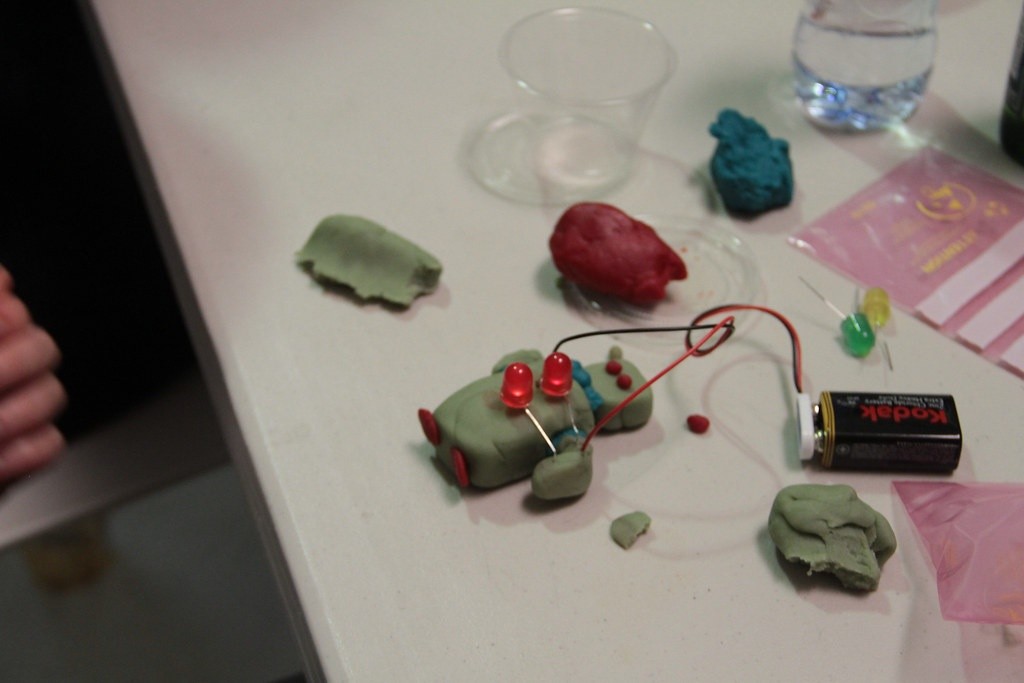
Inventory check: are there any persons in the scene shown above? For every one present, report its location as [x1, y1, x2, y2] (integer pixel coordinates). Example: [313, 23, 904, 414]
[0, 264, 69, 485]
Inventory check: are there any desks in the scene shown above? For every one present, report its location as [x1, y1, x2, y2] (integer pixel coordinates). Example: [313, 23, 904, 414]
[83, 0, 1023, 681]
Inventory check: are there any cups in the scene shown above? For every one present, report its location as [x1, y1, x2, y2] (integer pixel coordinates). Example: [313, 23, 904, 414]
[790, 0, 939, 133]
[496, 5, 678, 190]
